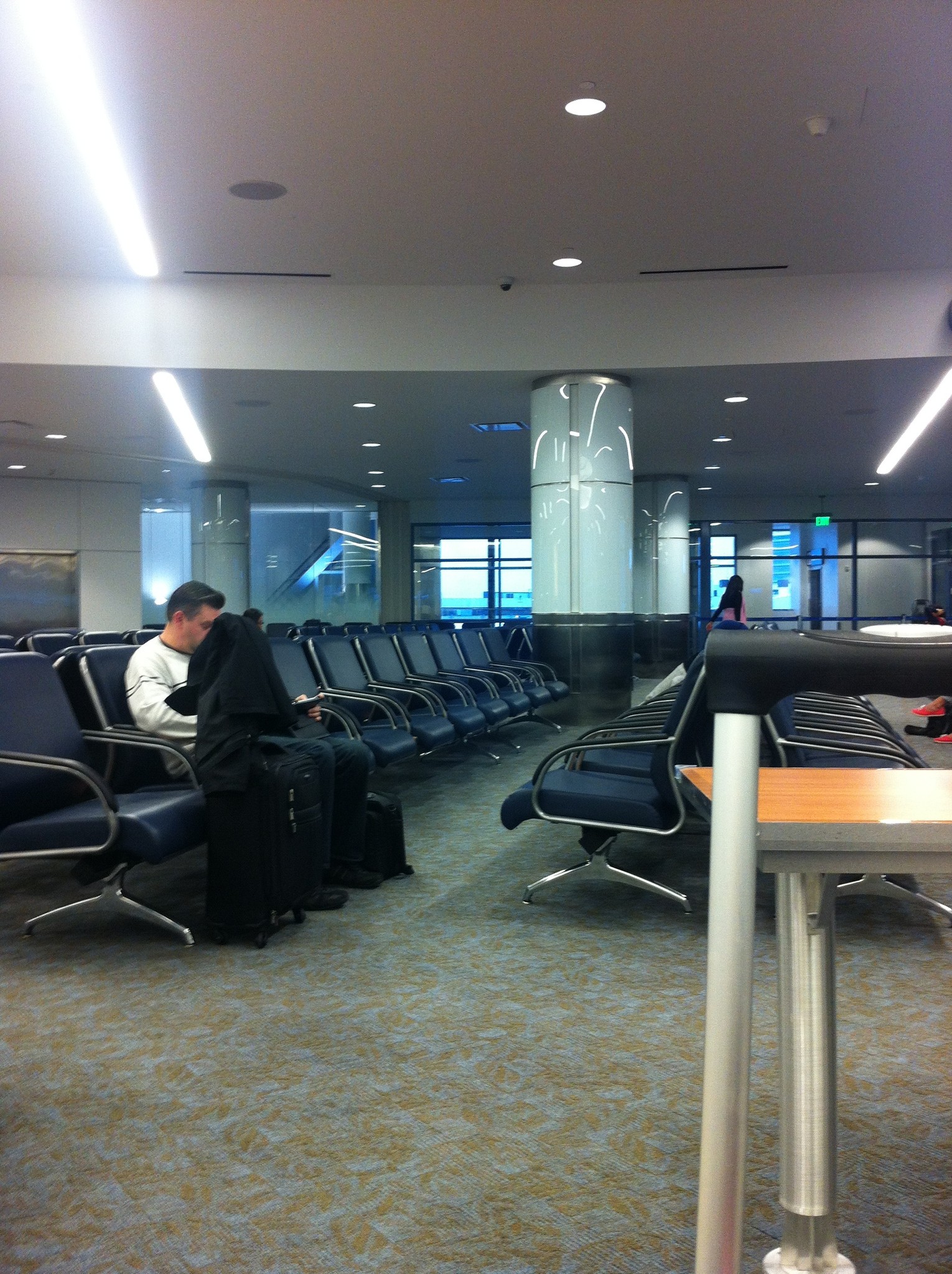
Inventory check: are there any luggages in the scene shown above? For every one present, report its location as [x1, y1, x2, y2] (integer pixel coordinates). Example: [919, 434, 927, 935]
[205, 750, 324, 947]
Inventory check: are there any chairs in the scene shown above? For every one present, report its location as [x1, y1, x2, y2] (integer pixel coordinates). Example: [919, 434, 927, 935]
[0, 616, 952, 950]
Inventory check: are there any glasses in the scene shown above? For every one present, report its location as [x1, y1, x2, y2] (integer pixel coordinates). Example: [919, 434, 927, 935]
[257, 621, 264, 625]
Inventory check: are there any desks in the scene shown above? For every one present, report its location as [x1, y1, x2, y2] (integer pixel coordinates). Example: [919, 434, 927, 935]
[671, 761, 950, 1274]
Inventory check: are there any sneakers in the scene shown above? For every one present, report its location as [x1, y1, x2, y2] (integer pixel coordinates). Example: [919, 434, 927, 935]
[911, 705, 945, 717]
[933, 736, 952, 743]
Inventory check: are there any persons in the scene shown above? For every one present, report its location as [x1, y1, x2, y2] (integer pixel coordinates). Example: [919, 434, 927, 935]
[923, 604, 948, 624]
[704, 574, 747, 630]
[910, 683, 952, 744]
[242, 607, 265, 630]
[122, 580, 383, 912]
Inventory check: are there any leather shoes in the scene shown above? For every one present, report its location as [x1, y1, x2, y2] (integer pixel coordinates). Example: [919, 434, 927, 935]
[301, 888, 348, 912]
[342, 870, 382, 888]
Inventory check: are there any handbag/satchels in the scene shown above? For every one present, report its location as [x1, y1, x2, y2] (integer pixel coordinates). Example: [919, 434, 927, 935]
[334, 792, 405, 877]
